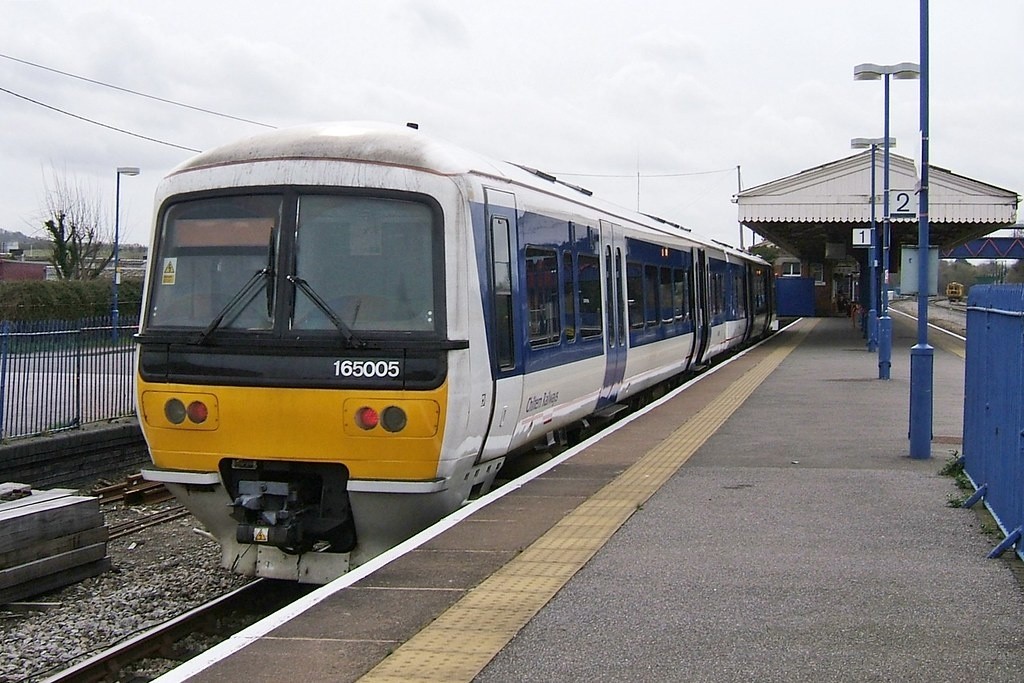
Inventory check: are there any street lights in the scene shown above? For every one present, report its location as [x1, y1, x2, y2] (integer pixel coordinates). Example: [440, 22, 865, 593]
[111, 167, 142, 346]
[851, 137, 898, 351]
[852, 62, 920, 378]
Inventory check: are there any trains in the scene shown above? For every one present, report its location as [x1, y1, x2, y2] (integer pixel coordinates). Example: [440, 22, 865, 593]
[945, 282, 965, 302]
[125, 122, 779, 588]
